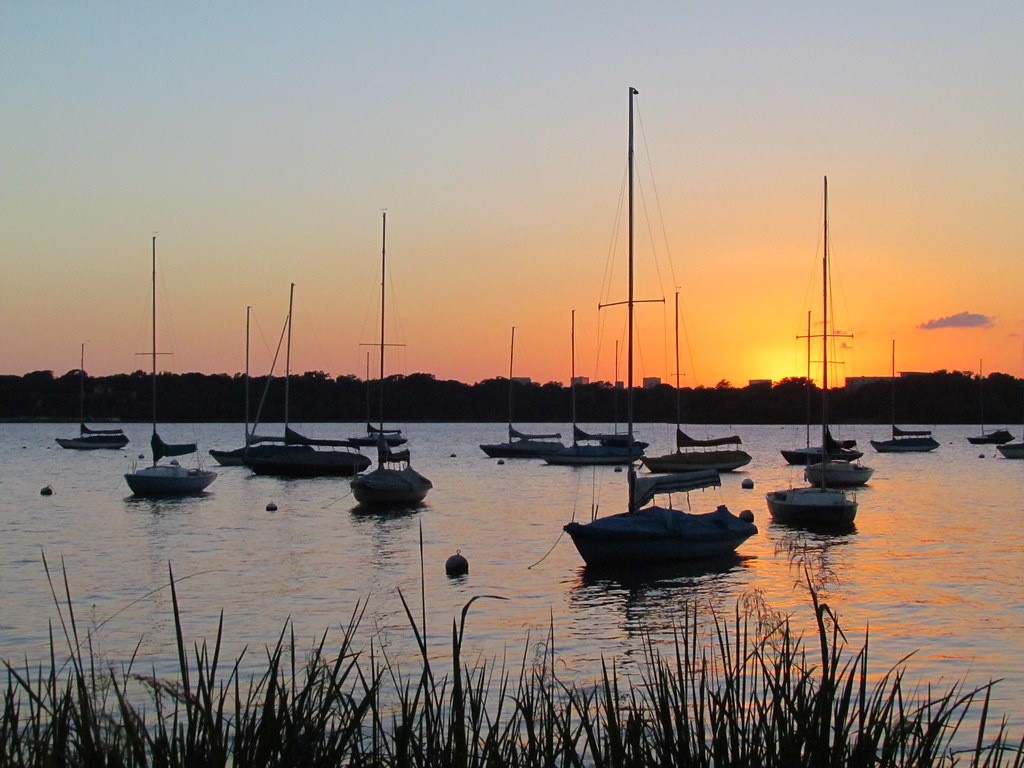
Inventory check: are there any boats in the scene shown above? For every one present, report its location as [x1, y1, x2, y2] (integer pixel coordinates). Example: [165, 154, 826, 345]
[801, 458, 875, 483]
[996, 438, 1024, 459]
[561, 470, 758, 566]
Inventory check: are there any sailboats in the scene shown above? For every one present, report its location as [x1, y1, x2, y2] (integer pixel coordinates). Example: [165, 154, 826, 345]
[869, 339, 941, 452]
[54, 344, 130, 449]
[778, 309, 863, 464]
[247, 288, 371, 476]
[765, 172, 858, 528]
[544, 310, 643, 463]
[122, 236, 218, 496]
[641, 291, 755, 470]
[594, 85, 652, 450]
[346, 213, 432, 505]
[479, 327, 566, 458]
[209, 306, 256, 468]
[349, 351, 408, 445]
[967, 358, 1016, 445]
